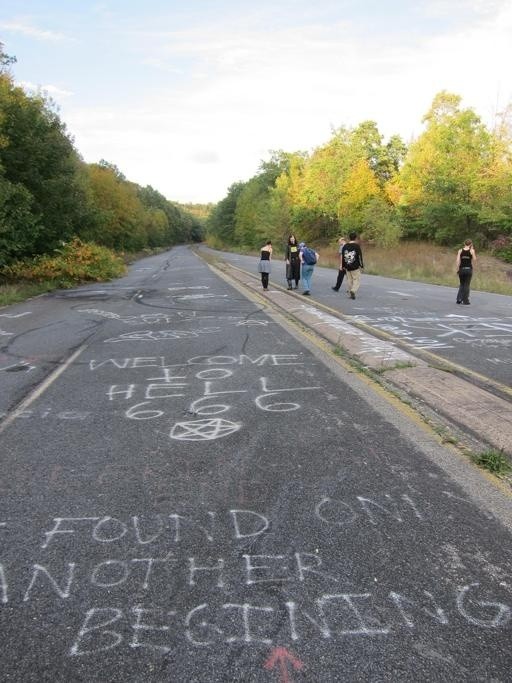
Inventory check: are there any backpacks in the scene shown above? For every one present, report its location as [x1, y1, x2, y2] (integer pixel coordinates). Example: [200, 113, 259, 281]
[303, 248, 317, 265]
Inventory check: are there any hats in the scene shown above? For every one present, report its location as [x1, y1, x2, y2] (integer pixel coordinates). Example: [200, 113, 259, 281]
[297, 242, 305, 249]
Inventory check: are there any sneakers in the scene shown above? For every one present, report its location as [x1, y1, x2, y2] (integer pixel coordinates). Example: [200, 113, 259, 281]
[330, 286, 356, 300]
[263, 288, 269, 291]
[287, 285, 312, 295]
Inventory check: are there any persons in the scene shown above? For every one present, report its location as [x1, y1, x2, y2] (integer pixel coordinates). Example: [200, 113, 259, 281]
[284, 234, 300, 290]
[298, 241, 319, 295]
[455, 238, 476, 304]
[331, 237, 347, 290]
[342, 232, 364, 299]
[258, 240, 272, 290]
